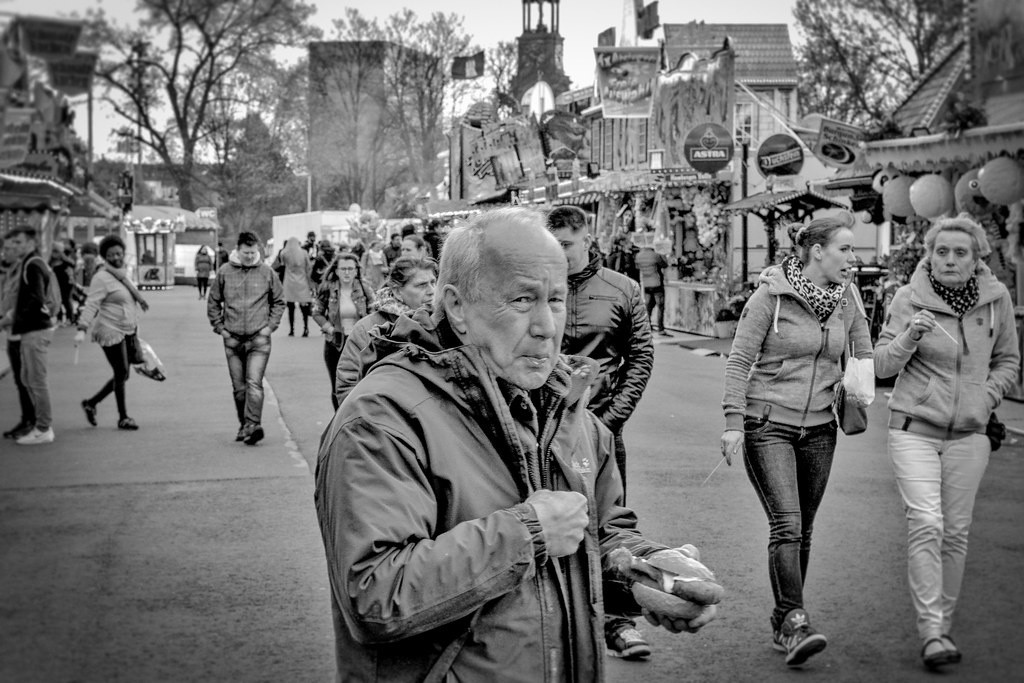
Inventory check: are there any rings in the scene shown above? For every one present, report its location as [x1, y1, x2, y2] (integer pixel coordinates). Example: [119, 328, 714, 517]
[914, 318, 919, 325]
[721, 447, 724, 448]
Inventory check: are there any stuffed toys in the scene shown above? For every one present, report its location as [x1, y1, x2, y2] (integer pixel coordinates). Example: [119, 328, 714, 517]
[870, 157, 1023, 265]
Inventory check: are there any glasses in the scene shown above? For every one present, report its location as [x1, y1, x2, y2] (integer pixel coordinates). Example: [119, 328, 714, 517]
[337, 266, 354, 271]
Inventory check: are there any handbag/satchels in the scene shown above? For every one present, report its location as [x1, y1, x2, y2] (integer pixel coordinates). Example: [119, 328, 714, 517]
[986, 412, 1006, 451]
[834, 292, 868, 435]
[126, 334, 168, 382]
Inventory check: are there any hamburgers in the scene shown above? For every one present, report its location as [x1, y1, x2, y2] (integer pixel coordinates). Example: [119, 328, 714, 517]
[610, 547, 725, 620]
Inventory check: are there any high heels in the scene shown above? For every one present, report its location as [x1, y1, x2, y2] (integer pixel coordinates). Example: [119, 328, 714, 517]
[301, 330, 308, 336]
[288, 330, 294, 335]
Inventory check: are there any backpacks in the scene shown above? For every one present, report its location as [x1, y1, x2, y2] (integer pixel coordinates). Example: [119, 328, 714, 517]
[23, 256, 62, 316]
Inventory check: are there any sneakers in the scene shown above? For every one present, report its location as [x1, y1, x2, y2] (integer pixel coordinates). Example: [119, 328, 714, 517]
[772, 631, 787, 652]
[15, 427, 55, 445]
[784, 608, 827, 665]
[603, 618, 651, 658]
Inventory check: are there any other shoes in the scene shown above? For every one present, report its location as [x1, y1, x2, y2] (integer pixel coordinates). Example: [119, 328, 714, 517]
[3, 420, 35, 439]
[660, 331, 673, 337]
[940, 634, 962, 664]
[243, 421, 264, 444]
[920, 638, 948, 668]
[82, 399, 97, 426]
[118, 418, 139, 430]
[235, 428, 245, 441]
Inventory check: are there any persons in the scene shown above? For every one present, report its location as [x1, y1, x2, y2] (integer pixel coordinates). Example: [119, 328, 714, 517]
[0, 226, 103, 445]
[720, 219, 875, 664]
[875, 217, 1020, 664]
[75, 235, 148, 430]
[310, 211, 719, 683]
[196, 207, 667, 460]
